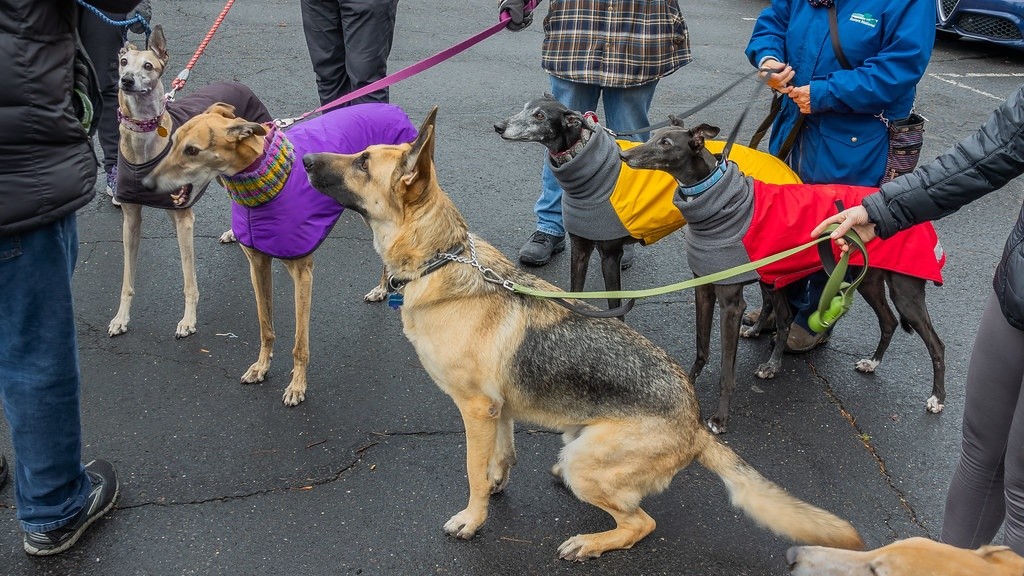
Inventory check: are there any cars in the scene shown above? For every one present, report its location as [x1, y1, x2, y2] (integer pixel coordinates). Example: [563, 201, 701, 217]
[933, 0, 1024, 58]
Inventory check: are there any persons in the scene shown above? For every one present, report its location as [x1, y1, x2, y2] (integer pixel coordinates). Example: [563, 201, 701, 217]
[809, 80, 1024, 561]
[0, 0, 138, 560]
[499, 0, 694, 273]
[296, 0, 402, 114]
[737, 0, 939, 354]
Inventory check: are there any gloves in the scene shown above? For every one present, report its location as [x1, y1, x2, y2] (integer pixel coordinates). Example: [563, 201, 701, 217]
[74, 58, 93, 133]
[126, 0, 153, 34]
[498, 0, 534, 32]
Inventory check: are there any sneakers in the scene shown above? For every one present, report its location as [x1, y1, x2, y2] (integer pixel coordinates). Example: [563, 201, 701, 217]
[741, 309, 776, 334]
[0, 451, 9, 493]
[620, 243, 634, 269]
[770, 321, 833, 356]
[23, 458, 119, 555]
[518, 229, 567, 265]
[106, 165, 121, 206]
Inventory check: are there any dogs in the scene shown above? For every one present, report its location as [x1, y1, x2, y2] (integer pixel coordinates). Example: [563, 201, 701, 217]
[112, 23, 419, 406]
[495, 91, 948, 433]
[785, 535, 1020, 575]
[303, 105, 860, 560]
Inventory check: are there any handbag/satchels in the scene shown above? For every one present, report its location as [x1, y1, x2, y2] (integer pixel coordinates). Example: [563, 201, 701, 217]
[879, 112, 929, 188]
